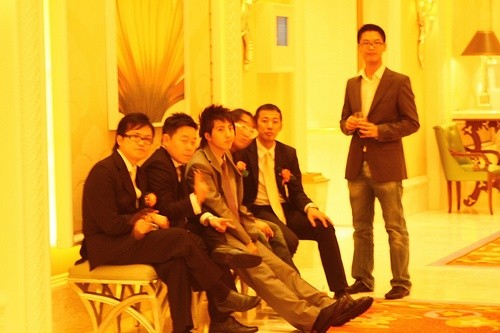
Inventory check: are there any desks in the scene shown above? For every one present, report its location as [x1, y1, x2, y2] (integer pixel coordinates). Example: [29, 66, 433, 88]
[452, 110, 500, 206]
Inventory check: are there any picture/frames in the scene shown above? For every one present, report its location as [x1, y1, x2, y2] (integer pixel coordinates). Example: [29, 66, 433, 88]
[106, 0, 193, 131]
[277, 16, 287, 45]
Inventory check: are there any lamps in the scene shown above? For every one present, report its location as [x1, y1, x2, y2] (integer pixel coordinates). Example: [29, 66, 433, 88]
[460, 30, 500, 110]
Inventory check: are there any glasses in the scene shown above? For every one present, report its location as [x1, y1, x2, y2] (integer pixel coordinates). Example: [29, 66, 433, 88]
[235, 124, 259, 138]
[124, 134, 154, 145]
[358, 41, 385, 50]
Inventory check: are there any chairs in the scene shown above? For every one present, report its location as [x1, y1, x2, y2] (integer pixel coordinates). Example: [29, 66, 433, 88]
[433, 122, 500, 215]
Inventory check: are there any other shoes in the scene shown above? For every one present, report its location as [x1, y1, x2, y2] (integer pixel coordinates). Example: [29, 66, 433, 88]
[208, 316, 258, 333]
[348, 279, 374, 294]
[215, 290, 261, 313]
[331, 297, 373, 327]
[312, 294, 347, 333]
[334, 292, 354, 300]
[385, 286, 411, 300]
[211, 244, 263, 269]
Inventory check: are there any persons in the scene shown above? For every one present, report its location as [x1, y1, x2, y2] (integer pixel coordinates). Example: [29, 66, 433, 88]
[234, 104, 352, 300]
[82, 112, 262, 333]
[340, 23, 420, 300]
[139, 103, 373, 333]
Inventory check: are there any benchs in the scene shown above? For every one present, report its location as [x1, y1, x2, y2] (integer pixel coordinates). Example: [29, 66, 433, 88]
[68, 259, 248, 333]
